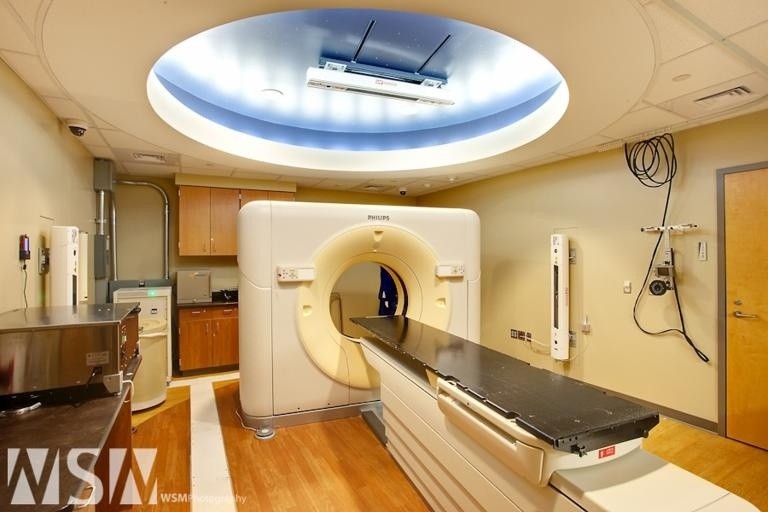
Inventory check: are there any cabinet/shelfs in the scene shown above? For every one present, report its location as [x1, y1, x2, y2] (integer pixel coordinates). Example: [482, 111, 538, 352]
[178, 184, 240, 256]
[240, 188, 296, 209]
[179, 306, 239, 377]
[113, 286, 173, 387]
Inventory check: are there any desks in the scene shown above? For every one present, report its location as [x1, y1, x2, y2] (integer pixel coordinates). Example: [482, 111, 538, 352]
[0, 353, 143, 512]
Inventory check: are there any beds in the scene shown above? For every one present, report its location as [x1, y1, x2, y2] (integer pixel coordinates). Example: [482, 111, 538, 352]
[348, 312, 764, 512]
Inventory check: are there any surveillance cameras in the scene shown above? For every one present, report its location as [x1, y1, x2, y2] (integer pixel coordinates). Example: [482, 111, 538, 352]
[67, 119, 88, 137]
